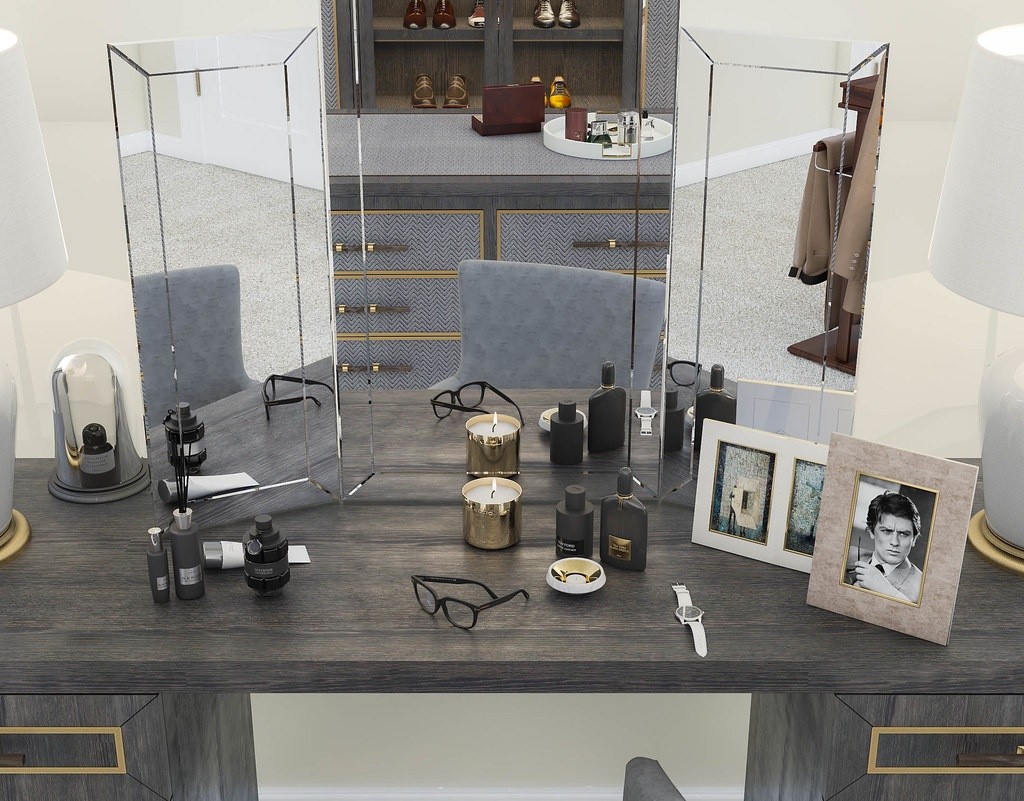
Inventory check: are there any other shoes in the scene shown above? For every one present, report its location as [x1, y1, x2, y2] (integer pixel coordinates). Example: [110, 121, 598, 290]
[468, 2, 487, 28]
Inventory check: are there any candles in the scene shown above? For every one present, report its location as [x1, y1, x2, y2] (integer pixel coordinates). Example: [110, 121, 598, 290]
[460, 478, 522, 550]
[466, 413, 521, 478]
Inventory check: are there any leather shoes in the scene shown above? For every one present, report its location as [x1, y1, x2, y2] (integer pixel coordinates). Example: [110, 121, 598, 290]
[443, 74, 471, 108]
[530, 76, 548, 108]
[432, 0, 456, 29]
[403, 0, 427, 29]
[550, 76, 571, 108]
[558, 0, 581, 28]
[533, 0, 555, 28]
[412, 74, 437, 108]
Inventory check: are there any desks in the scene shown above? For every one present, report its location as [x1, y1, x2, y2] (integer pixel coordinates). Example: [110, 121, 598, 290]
[147, 354, 338, 529]
[337, 388, 665, 502]
[0, 458, 1023, 801]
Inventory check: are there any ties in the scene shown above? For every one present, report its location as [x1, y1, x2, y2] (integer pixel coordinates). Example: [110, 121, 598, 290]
[875, 564, 884, 572]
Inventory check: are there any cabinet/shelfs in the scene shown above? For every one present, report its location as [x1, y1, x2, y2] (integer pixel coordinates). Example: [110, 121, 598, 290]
[318, 1, 680, 391]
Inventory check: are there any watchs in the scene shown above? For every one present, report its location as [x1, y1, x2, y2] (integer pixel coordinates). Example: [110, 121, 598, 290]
[670, 582, 708, 658]
[635, 390, 659, 437]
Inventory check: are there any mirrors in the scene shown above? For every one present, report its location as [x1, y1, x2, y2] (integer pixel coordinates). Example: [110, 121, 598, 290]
[109, 0, 886, 534]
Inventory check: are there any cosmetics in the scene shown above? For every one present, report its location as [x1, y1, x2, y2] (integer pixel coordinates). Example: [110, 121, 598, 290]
[555, 484, 595, 557]
[600, 467, 648, 571]
[565, 107, 655, 149]
[549, 360, 627, 464]
[147, 507, 312, 604]
[79, 401, 259, 503]
[665, 364, 736, 453]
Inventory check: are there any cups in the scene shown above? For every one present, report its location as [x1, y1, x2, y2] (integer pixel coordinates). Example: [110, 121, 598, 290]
[565, 107, 587, 142]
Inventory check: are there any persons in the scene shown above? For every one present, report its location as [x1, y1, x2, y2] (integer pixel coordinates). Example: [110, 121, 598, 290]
[847, 492, 923, 604]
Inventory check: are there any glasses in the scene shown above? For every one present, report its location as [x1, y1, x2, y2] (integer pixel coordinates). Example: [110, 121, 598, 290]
[411, 574, 529, 630]
[430, 380, 524, 426]
[847, 536, 862, 575]
[666, 360, 701, 386]
[262, 374, 334, 420]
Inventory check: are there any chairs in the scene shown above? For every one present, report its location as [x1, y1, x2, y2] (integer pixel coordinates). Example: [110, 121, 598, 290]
[134, 265, 263, 431]
[431, 253, 667, 393]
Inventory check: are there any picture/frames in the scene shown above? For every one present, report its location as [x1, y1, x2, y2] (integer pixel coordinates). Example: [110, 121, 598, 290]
[806, 433, 980, 647]
